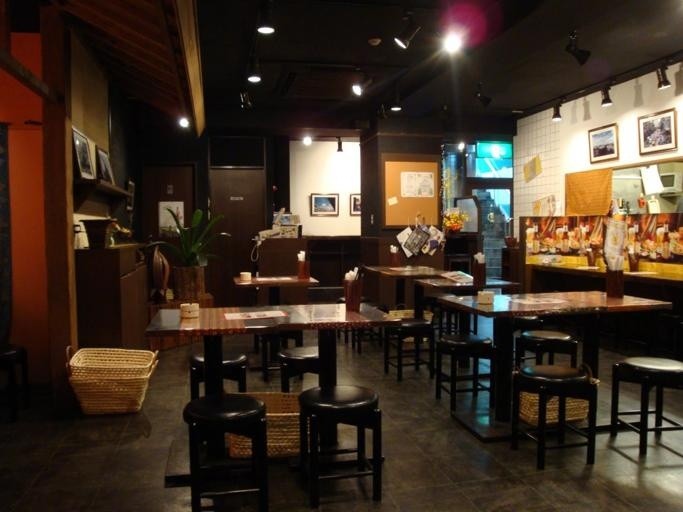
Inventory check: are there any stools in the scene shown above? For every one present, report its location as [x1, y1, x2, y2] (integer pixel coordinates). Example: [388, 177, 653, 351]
[609, 356, 683, 467]
[190, 350, 249, 399]
[382, 316, 436, 378]
[278, 347, 320, 394]
[435, 335, 492, 418]
[253, 330, 303, 384]
[515, 329, 578, 367]
[297, 385, 383, 511]
[181, 394, 269, 511]
[510, 364, 600, 471]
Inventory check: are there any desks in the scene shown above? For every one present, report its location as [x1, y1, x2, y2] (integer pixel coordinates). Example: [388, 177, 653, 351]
[530, 261, 682, 287]
[233, 276, 319, 305]
[438, 291, 669, 443]
[364, 264, 449, 347]
[145, 302, 403, 486]
[418, 275, 519, 368]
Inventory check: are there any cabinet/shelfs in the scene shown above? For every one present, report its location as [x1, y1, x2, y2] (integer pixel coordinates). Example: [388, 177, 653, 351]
[75, 243, 146, 353]
[256, 234, 313, 306]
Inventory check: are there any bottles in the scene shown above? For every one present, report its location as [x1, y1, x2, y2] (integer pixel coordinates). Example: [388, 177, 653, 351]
[525, 215, 678, 271]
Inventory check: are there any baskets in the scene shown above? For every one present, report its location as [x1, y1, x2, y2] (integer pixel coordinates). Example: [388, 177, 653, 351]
[65, 347, 160, 417]
[519, 378, 598, 427]
[228, 390, 305, 460]
[390, 308, 433, 343]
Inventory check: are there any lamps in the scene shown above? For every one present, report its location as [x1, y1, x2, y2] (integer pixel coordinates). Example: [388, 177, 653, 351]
[562, 20, 595, 68]
[349, 2, 447, 117]
[516, 50, 682, 122]
[471, 77, 497, 112]
[245, 0, 281, 94]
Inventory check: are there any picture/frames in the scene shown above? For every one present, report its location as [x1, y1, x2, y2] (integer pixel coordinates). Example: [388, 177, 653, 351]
[71, 127, 94, 180]
[310, 192, 339, 217]
[350, 193, 361, 217]
[95, 145, 114, 185]
[637, 107, 677, 155]
[587, 122, 617, 163]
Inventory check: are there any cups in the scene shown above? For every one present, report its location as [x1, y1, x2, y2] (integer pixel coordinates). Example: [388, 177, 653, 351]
[502, 217, 520, 249]
[297, 261, 311, 283]
[471, 260, 487, 295]
[390, 251, 399, 267]
[605, 264, 625, 303]
[476, 291, 494, 306]
[343, 279, 363, 313]
[239, 280, 251, 284]
[405, 264, 434, 274]
[239, 271, 251, 280]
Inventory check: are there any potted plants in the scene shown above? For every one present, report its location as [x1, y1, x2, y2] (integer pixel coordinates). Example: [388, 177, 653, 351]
[149, 207, 231, 297]
